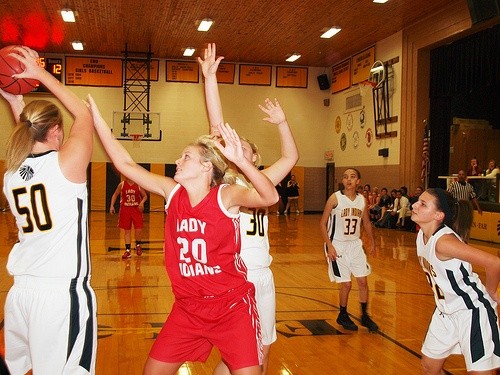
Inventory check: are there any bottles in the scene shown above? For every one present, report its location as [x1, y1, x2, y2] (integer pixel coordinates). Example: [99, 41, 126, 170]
[483, 168, 486, 176]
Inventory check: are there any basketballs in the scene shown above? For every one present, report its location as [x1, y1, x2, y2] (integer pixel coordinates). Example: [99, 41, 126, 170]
[0, 44, 41, 95]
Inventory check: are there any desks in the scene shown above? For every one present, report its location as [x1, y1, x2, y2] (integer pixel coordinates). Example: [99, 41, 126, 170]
[438, 173, 497, 202]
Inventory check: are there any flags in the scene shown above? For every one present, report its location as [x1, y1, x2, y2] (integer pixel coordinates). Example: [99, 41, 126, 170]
[420, 117, 431, 191]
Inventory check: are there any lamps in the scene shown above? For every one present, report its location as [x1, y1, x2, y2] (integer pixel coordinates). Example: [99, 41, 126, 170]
[286, 52, 303, 63]
[72, 40, 84, 51]
[320, 25, 341, 39]
[60, 8, 77, 24]
[196, 18, 215, 32]
[181, 47, 198, 58]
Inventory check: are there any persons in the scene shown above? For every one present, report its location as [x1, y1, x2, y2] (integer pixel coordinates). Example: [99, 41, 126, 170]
[446, 159, 500, 243]
[275, 175, 301, 214]
[409, 186, 500, 375]
[0, 45, 97, 375]
[357, 184, 431, 233]
[110, 174, 147, 259]
[319, 168, 378, 332]
[82, 93, 279, 375]
[198, 42, 300, 375]
[2, 199, 9, 211]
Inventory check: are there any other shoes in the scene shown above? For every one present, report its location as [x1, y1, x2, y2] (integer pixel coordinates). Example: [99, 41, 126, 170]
[122, 249, 132, 259]
[122, 259, 131, 269]
[359, 313, 378, 331]
[336, 312, 359, 330]
[134, 257, 142, 269]
[284, 210, 288, 214]
[296, 210, 300, 214]
[136, 244, 142, 255]
